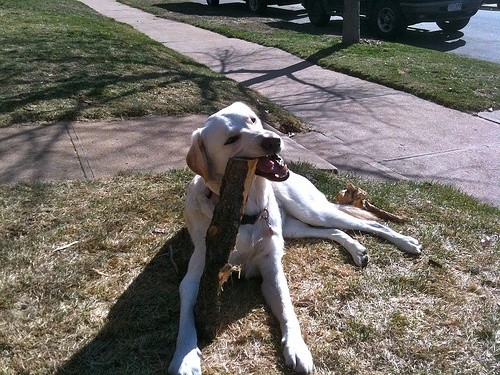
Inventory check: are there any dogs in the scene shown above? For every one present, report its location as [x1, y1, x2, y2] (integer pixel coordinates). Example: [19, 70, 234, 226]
[163, 101, 424, 375]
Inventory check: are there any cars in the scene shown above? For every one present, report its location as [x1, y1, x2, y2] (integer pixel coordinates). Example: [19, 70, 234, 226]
[207, 0, 303, 14]
[302, 0, 484, 41]
